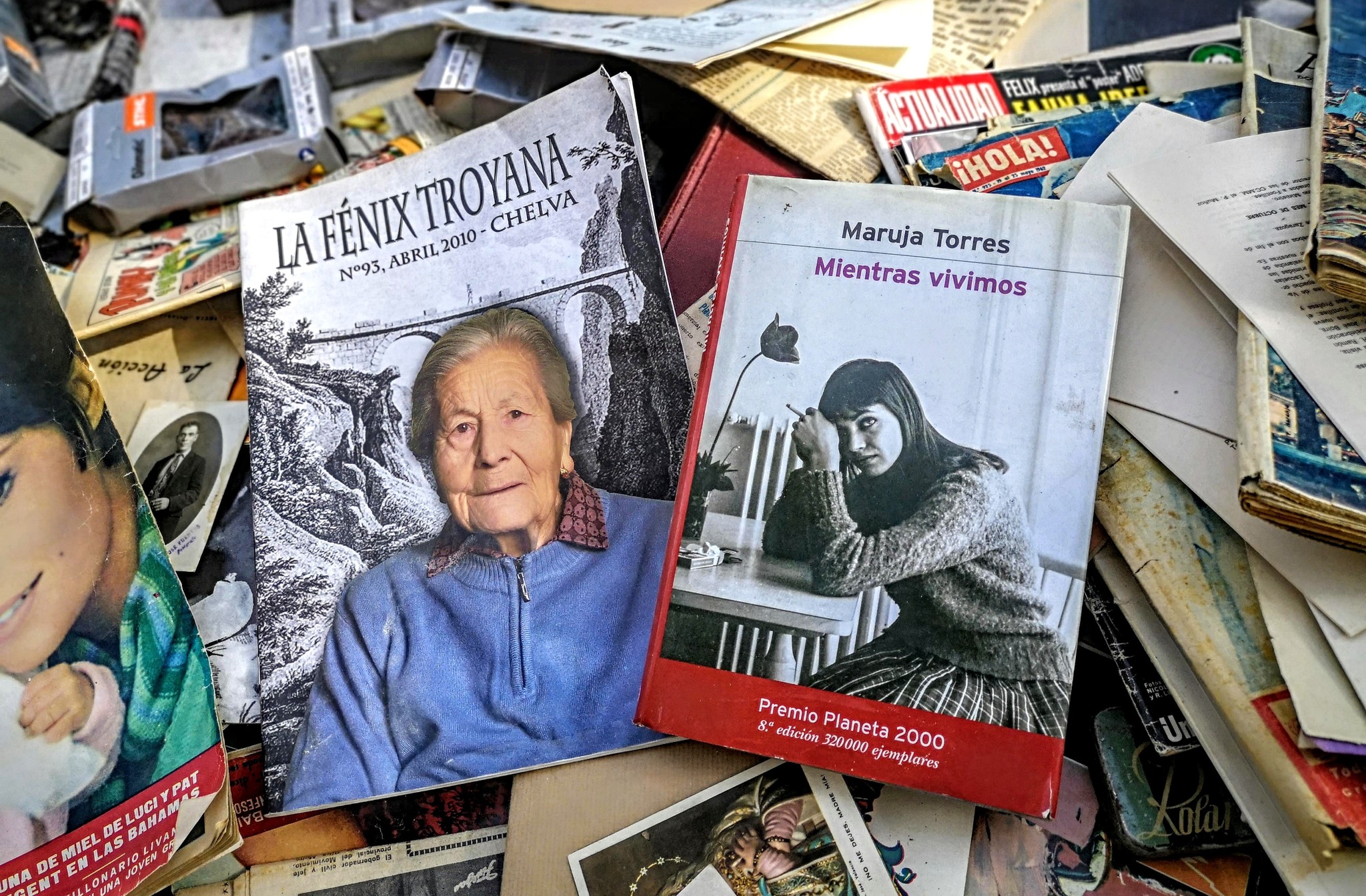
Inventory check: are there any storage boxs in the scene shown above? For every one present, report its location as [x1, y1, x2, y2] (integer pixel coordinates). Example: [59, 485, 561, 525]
[677, 551, 714, 569]
[0, 0, 558, 237]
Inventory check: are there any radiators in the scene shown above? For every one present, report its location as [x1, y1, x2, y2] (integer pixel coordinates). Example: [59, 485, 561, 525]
[716, 412, 891, 685]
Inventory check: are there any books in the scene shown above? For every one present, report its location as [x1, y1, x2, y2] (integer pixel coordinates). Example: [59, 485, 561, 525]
[0, 0, 1366, 896]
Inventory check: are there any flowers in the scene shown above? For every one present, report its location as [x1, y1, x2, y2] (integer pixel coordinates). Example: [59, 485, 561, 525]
[692, 312, 800, 490]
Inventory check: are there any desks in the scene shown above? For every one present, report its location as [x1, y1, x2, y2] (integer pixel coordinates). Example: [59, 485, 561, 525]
[672, 511, 864, 685]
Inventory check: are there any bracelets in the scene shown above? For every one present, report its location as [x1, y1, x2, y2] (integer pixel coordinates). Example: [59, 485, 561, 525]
[766, 837, 790, 842]
[753, 845, 770, 869]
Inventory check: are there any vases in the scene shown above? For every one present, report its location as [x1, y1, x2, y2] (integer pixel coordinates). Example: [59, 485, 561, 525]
[682, 491, 712, 540]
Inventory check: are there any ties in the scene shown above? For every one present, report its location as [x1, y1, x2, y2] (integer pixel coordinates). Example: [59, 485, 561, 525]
[147, 455, 180, 508]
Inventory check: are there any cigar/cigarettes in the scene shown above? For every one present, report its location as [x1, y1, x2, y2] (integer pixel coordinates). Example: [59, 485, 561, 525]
[152, 502, 155, 505]
[787, 402, 804, 417]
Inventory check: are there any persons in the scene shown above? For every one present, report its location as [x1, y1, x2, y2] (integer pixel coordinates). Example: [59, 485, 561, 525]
[0, 659, 126, 866]
[0, 201, 221, 830]
[658, 768, 863, 896]
[282, 310, 674, 812]
[140, 418, 205, 545]
[763, 360, 1073, 736]
